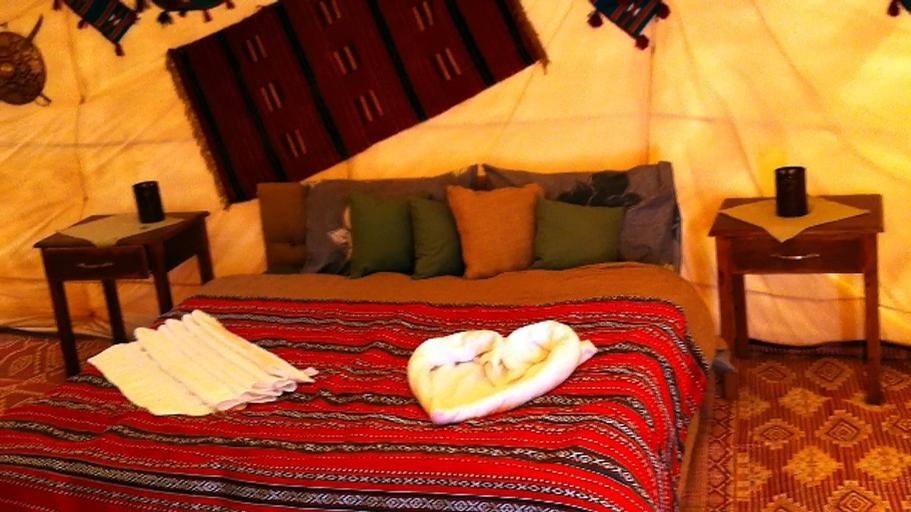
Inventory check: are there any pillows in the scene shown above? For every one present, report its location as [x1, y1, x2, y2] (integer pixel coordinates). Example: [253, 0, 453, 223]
[483, 164, 678, 270]
[445, 183, 546, 280]
[297, 176, 476, 275]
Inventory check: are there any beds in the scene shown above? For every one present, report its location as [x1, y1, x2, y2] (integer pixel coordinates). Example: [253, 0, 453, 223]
[1, 270, 718, 512]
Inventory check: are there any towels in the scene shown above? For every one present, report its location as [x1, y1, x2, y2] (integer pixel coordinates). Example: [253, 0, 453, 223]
[87, 307, 320, 416]
[402, 319, 599, 426]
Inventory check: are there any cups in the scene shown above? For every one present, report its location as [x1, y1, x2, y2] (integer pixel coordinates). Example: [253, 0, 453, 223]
[132, 181, 166, 225]
[774, 165, 810, 218]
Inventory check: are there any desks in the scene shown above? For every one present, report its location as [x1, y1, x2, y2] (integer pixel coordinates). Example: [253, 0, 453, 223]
[32, 210, 217, 376]
[708, 194, 886, 405]
